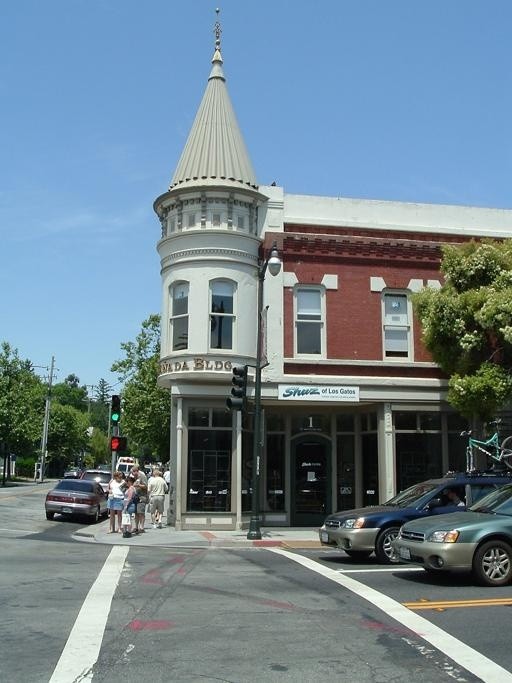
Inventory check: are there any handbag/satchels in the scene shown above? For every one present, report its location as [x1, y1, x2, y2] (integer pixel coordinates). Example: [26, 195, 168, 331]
[121, 512, 133, 526]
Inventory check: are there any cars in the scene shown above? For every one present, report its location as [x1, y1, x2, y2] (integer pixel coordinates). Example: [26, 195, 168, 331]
[319, 476, 512, 587]
[45, 479, 108, 520]
[64, 464, 112, 497]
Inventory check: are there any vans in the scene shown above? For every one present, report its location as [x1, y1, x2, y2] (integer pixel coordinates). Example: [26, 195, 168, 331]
[115, 457, 162, 479]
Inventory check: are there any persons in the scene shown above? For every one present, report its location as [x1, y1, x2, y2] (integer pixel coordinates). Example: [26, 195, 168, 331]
[444, 488, 465, 507]
[107, 465, 168, 538]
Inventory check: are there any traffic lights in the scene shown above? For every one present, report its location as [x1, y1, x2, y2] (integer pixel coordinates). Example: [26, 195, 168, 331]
[110, 436, 127, 453]
[225, 367, 248, 415]
[111, 395, 121, 427]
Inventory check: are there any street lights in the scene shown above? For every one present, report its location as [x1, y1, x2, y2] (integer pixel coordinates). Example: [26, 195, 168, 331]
[247, 240, 281, 540]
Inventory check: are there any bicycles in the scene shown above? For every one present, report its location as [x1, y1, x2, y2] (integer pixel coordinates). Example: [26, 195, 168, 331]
[460, 419, 512, 475]
